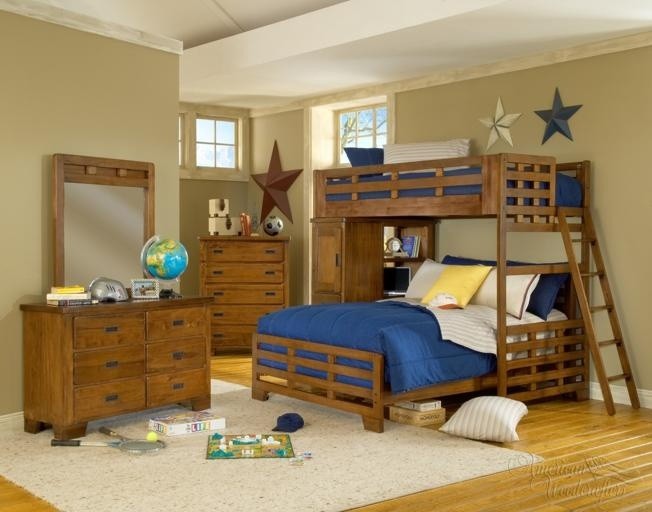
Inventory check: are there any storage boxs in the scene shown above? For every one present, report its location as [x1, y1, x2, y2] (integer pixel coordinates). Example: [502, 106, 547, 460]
[392, 398, 441, 412]
[389, 406, 446, 427]
[147, 409, 226, 436]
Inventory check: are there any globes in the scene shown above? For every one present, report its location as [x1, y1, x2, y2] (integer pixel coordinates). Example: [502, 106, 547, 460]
[140, 235, 188, 298]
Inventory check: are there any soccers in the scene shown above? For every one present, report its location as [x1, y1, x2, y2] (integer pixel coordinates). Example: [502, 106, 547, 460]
[263, 215, 283, 235]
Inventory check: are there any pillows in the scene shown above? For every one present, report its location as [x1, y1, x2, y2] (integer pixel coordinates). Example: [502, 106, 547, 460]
[405, 257, 468, 307]
[382, 137, 471, 177]
[504, 260, 569, 320]
[439, 396, 529, 442]
[420, 264, 493, 310]
[442, 254, 508, 266]
[343, 147, 383, 177]
[469, 260, 541, 320]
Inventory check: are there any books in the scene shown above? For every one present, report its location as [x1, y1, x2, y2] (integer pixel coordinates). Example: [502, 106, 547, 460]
[402, 236, 420, 258]
[51, 285, 84, 293]
[47, 291, 92, 300]
[47, 299, 91, 307]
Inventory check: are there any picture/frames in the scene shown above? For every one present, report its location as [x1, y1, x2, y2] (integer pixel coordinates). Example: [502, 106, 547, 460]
[132, 278, 160, 300]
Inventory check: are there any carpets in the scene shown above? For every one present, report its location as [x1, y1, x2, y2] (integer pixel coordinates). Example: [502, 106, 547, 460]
[2, 377, 543, 510]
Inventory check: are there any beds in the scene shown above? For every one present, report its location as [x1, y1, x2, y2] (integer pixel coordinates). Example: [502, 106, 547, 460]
[251, 152, 642, 433]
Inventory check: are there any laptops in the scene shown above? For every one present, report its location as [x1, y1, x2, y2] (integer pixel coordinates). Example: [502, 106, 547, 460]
[384, 266, 411, 297]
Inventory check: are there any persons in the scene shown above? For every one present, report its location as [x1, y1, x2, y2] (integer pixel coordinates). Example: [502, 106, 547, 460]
[139, 285, 146, 296]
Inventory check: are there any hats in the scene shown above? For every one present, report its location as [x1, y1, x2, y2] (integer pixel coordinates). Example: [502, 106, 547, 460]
[272, 413, 304, 432]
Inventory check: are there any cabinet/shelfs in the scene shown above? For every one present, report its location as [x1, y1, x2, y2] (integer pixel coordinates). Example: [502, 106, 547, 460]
[17, 290, 216, 439]
[198, 234, 292, 356]
